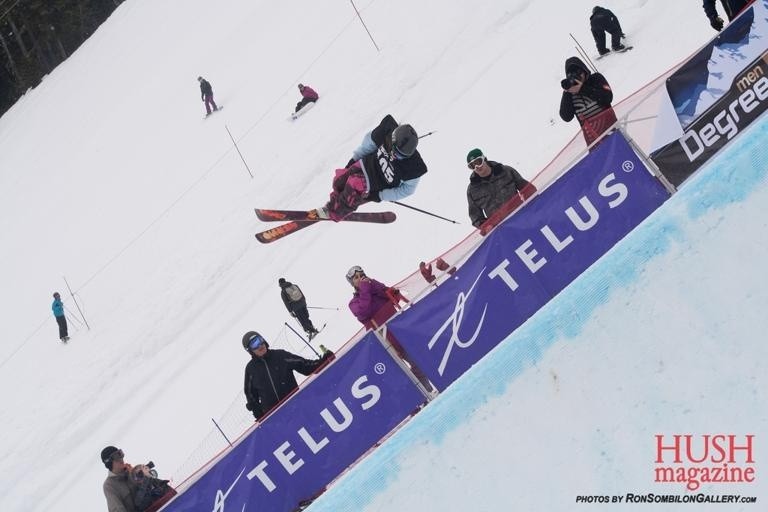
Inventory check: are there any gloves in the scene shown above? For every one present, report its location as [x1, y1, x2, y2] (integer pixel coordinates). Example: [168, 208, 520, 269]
[420, 262, 436, 283]
[290, 310, 298, 319]
[323, 352, 335, 362]
[436, 258, 456, 273]
[711, 18, 725, 32]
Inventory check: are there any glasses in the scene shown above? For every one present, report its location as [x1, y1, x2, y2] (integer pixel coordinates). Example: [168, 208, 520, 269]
[468, 156, 484, 170]
[110, 449, 125, 462]
[347, 266, 361, 277]
[248, 336, 264, 350]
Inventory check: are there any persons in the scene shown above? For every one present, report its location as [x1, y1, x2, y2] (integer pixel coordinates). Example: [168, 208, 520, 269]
[291, 83, 318, 121]
[241, 330, 336, 422]
[344, 265, 438, 407]
[702, 0, 752, 32]
[559, 56, 618, 156]
[100, 445, 143, 511]
[197, 76, 218, 116]
[278, 277, 318, 334]
[589, 6, 626, 56]
[129, 463, 179, 512]
[465, 148, 538, 238]
[51, 291, 70, 344]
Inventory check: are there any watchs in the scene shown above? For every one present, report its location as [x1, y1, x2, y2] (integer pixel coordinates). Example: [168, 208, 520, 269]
[306, 112, 429, 224]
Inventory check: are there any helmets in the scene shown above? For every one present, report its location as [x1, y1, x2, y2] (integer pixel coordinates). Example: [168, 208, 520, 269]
[131, 465, 153, 483]
[100, 446, 119, 470]
[393, 124, 418, 156]
[242, 331, 269, 353]
[467, 149, 487, 162]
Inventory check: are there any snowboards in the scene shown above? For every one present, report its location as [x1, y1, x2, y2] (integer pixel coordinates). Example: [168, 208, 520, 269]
[286, 102, 314, 119]
[202, 107, 224, 118]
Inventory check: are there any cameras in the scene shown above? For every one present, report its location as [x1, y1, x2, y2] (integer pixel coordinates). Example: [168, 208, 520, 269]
[560, 71, 582, 90]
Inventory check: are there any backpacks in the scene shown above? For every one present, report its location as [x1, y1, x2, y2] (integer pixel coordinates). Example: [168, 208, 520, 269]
[285, 285, 302, 302]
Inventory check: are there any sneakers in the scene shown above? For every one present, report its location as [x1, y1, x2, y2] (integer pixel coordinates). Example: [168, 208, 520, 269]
[315, 207, 330, 221]
[600, 45, 624, 54]
